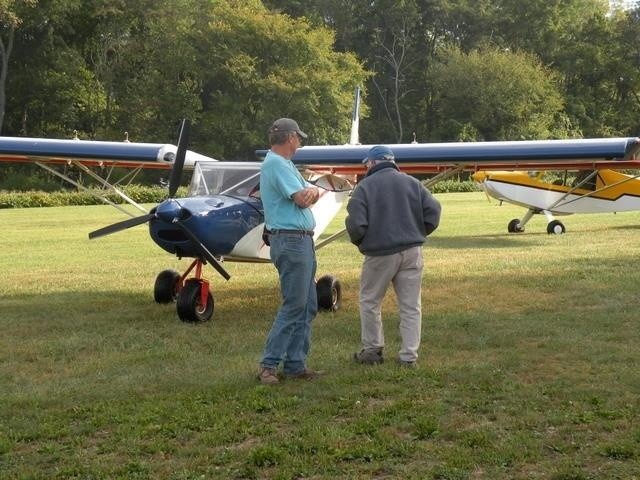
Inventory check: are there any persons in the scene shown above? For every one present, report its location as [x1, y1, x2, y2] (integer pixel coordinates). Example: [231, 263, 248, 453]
[253, 118, 325, 386]
[342, 144, 443, 373]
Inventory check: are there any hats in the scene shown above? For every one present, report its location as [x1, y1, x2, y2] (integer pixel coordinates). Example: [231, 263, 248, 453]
[362, 146, 395, 164]
[270, 118, 308, 139]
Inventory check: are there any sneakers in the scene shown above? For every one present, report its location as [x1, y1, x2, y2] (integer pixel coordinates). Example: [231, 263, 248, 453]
[398, 356, 417, 368]
[258, 366, 280, 385]
[352, 349, 384, 364]
[284, 366, 325, 379]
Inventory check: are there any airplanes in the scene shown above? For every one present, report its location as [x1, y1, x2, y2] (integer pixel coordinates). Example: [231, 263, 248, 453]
[469, 168, 639, 235]
[0, 85, 639, 325]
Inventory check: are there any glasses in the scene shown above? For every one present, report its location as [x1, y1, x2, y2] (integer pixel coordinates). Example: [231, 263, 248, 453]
[365, 160, 370, 167]
[291, 134, 302, 143]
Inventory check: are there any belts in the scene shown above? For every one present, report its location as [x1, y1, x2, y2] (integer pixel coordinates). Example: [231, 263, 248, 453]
[268, 230, 315, 236]
